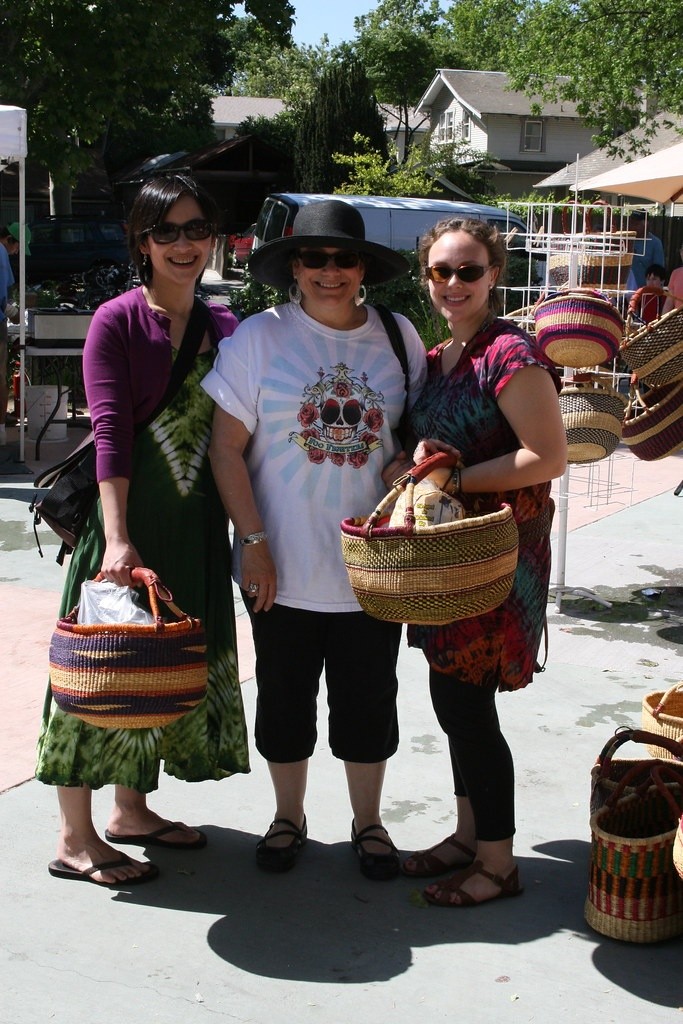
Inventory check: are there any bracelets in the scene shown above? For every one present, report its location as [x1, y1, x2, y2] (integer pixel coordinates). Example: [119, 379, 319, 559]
[441, 467, 461, 494]
[240, 531, 265, 546]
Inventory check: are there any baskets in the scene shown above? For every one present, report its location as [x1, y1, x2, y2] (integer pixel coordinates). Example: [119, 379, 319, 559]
[577, 350, 627, 387]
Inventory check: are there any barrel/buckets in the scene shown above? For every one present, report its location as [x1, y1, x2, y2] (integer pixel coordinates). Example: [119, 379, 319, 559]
[25, 386, 68, 440]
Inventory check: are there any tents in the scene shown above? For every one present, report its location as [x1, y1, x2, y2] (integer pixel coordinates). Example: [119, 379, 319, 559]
[0, 104, 27, 461]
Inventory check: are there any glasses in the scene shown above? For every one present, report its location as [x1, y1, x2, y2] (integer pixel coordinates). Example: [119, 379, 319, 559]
[139, 219, 212, 244]
[293, 249, 359, 269]
[425, 263, 496, 283]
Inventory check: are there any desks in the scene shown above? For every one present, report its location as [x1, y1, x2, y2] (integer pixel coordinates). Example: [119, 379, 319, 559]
[23, 344, 87, 462]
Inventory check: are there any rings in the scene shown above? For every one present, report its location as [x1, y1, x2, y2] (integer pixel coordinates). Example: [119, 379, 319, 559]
[249, 584, 258, 592]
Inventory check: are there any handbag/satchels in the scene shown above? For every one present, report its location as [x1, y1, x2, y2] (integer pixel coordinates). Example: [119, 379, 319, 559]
[28, 430, 98, 567]
[48, 565, 208, 728]
[620, 286, 683, 388]
[533, 289, 625, 368]
[340, 451, 520, 626]
[584, 680, 683, 944]
[622, 372, 683, 461]
[549, 200, 636, 298]
[557, 374, 627, 464]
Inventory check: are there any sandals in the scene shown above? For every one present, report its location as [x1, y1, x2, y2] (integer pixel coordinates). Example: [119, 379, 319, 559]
[255, 812, 307, 871]
[399, 833, 478, 879]
[350, 819, 401, 881]
[422, 861, 524, 907]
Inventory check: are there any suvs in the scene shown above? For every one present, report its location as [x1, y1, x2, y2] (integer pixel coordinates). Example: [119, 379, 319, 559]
[7, 213, 131, 288]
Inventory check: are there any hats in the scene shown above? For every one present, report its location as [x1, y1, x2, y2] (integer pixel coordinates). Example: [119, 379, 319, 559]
[248, 200, 410, 290]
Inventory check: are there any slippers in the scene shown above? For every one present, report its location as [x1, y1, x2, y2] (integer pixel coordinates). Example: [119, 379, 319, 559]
[105, 820, 206, 850]
[48, 850, 159, 885]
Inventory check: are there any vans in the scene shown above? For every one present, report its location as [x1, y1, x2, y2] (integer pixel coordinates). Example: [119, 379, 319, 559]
[248, 193, 548, 289]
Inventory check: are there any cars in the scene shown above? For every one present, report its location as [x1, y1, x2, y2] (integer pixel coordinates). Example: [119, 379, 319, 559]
[228, 222, 257, 268]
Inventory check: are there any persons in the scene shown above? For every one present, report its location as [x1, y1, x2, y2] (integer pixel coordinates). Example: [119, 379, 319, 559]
[661, 244, 683, 314]
[0, 223, 31, 444]
[623, 209, 665, 301]
[199, 201, 462, 881]
[639, 264, 667, 323]
[381, 216, 566, 908]
[34, 173, 250, 886]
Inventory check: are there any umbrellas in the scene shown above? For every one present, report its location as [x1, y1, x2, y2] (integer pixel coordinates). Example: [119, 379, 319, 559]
[569, 142, 683, 206]
